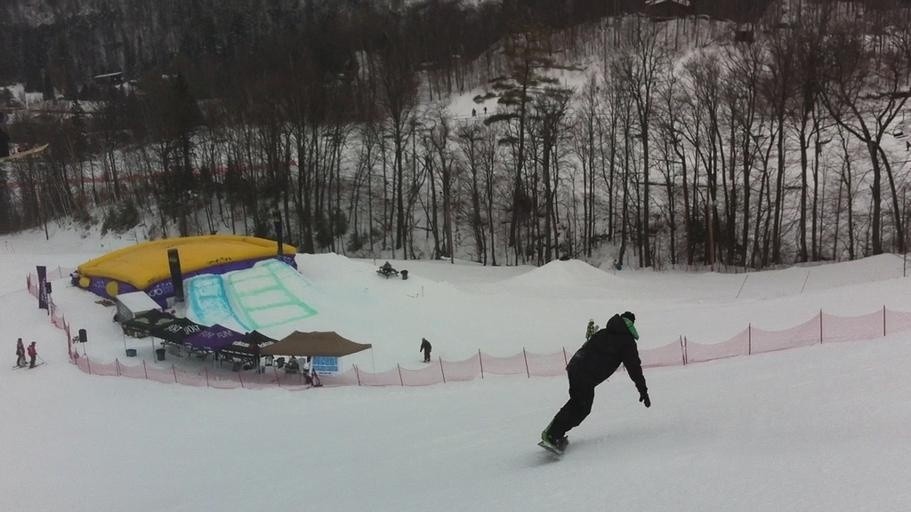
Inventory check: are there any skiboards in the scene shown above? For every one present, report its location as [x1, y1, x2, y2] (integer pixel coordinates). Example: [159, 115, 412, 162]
[11, 359, 44, 370]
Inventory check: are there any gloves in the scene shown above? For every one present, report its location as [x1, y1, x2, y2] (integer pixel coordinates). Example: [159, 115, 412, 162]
[639, 390, 651, 408]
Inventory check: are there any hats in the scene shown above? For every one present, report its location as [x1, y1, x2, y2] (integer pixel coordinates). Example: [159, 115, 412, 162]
[621, 311, 636, 325]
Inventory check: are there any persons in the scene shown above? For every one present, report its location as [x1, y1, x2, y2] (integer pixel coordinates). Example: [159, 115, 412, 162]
[585, 318, 595, 341]
[299, 355, 320, 388]
[284, 355, 297, 373]
[540, 309, 653, 452]
[27, 341, 37, 368]
[382, 260, 391, 274]
[15, 337, 28, 366]
[419, 338, 432, 363]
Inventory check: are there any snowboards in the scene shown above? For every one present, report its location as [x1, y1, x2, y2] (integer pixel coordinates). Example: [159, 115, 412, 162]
[539, 431, 569, 454]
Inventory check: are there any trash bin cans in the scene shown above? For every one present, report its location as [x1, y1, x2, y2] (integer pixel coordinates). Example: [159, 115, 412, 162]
[156, 349, 166, 361]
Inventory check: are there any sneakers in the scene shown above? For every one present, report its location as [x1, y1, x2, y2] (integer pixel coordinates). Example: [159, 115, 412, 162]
[542, 430, 570, 451]
